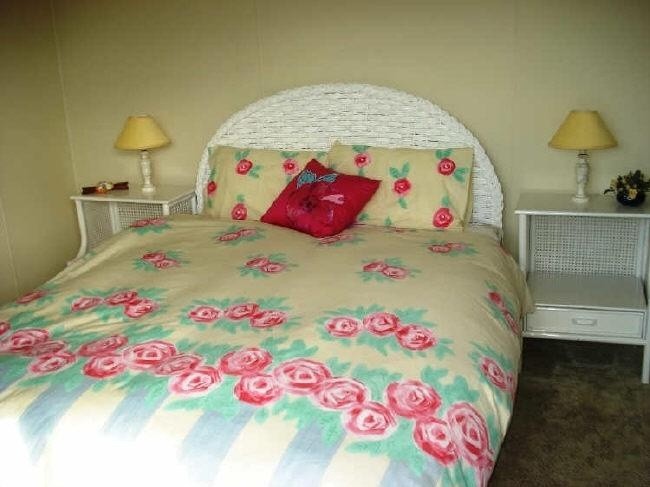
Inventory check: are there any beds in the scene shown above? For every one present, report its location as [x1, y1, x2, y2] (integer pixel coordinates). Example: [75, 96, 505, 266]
[2, 82, 509, 483]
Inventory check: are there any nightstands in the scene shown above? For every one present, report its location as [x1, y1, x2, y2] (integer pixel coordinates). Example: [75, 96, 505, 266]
[513, 189, 650, 385]
[66, 189, 196, 266]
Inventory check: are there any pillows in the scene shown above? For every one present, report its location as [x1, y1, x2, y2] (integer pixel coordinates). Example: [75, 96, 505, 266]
[211, 146, 323, 221]
[326, 143, 473, 230]
[261, 157, 381, 236]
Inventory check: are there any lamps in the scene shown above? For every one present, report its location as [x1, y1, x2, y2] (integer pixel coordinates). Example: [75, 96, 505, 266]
[549, 107, 618, 206]
[114, 114, 170, 190]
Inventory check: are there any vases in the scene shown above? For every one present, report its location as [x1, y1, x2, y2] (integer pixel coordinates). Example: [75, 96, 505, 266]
[618, 193, 646, 204]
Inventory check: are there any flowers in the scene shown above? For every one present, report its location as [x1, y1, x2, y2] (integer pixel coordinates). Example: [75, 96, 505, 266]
[602, 168, 650, 199]
